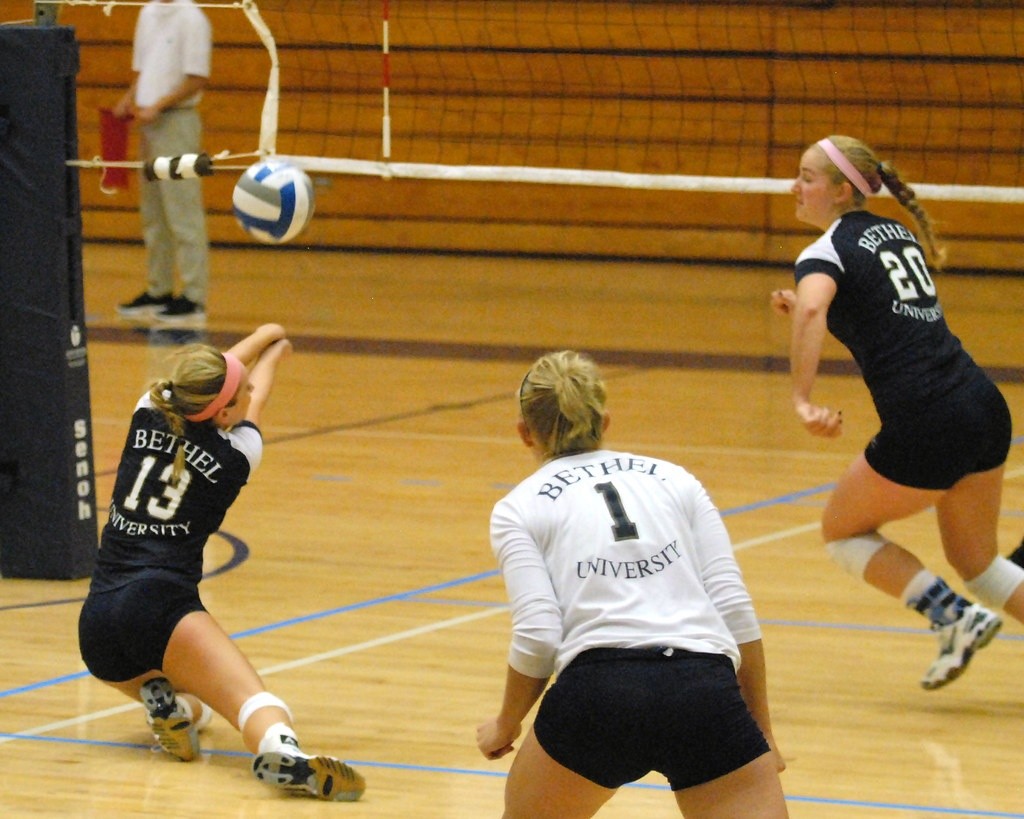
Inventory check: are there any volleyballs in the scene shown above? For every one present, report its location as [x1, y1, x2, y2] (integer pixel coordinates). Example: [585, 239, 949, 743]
[231, 158, 319, 247]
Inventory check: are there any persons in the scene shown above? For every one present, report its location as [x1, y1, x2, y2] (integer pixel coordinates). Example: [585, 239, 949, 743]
[77, 323, 367, 803]
[770, 135, 1023, 690]
[113, 1, 213, 322]
[475, 350, 791, 819]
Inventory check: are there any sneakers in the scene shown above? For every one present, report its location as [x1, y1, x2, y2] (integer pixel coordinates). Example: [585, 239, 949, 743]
[919, 603, 1001, 690]
[152, 294, 206, 322]
[252, 751, 366, 802]
[117, 291, 175, 316]
[139, 676, 199, 762]
